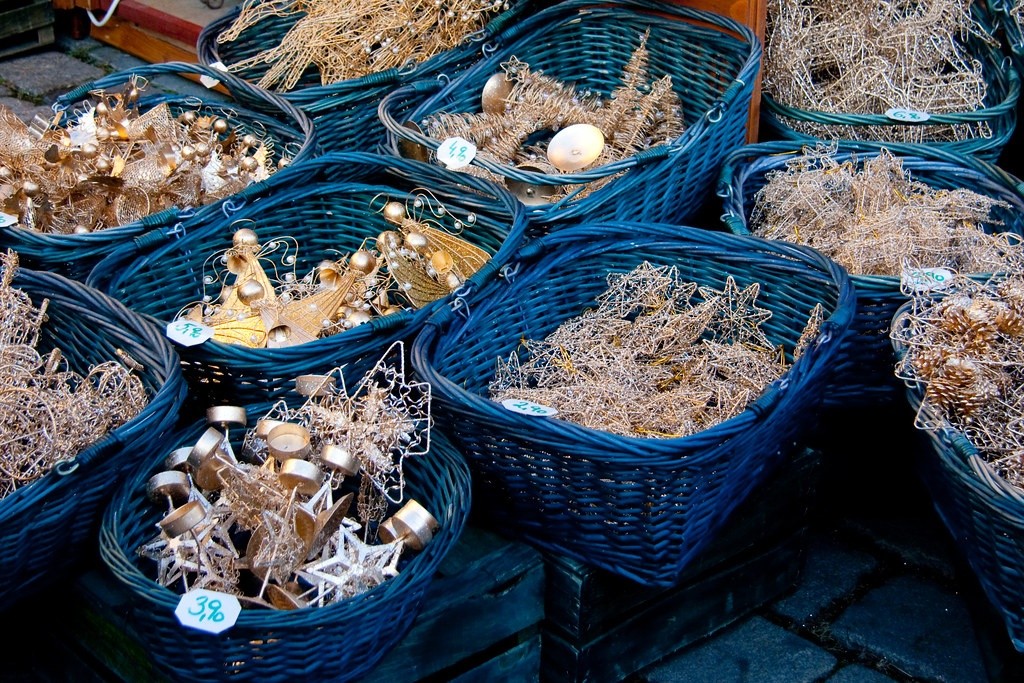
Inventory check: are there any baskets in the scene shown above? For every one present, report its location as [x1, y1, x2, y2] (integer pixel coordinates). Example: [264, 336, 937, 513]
[0, 0, 1024, 683]
